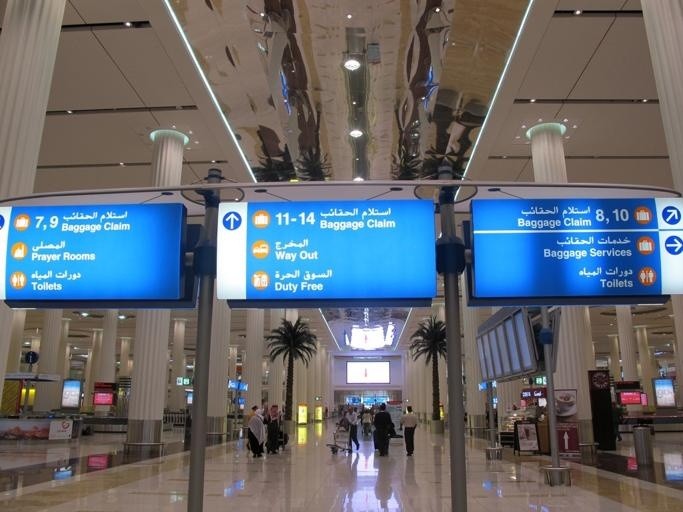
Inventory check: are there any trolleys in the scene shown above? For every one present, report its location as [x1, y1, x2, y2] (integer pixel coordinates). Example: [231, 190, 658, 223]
[276, 423, 285, 450]
[325, 422, 352, 455]
[372, 429, 389, 452]
[361, 422, 372, 434]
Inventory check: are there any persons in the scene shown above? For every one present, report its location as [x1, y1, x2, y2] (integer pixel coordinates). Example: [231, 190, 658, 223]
[264, 455, 282, 506]
[340, 402, 379, 439]
[247, 405, 264, 457]
[344, 453, 359, 502]
[245, 457, 263, 501]
[265, 404, 281, 455]
[341, 406, 359, 453]
[398, 405, 417, 456]
[374, 456, 393, 509]
[372, 402, 390, 456]
[361, 440, 372, 460]
[396, 456, 419, 505]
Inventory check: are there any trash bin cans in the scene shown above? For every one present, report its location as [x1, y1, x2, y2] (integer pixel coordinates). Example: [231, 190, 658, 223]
[632, 425, 654, 466]
